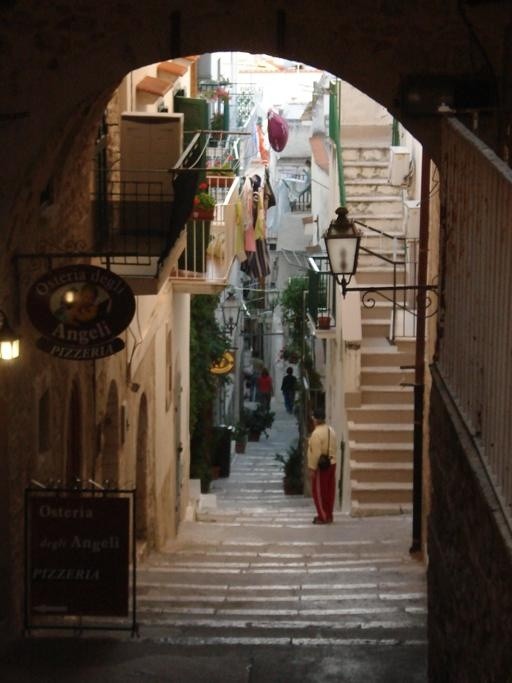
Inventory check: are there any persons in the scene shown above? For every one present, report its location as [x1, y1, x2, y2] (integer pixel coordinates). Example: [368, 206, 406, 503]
[257, 368, 273, 415]
[68, 285, 97, 324]
[282, 367, 297, 415]
[307, 410, 338, 524]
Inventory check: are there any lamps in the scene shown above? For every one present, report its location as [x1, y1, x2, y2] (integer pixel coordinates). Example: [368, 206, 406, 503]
[1, 323, 21, 360]
[323, 206, 364, 298]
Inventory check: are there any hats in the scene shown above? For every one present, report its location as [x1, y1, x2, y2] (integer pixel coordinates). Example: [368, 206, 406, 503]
[313, 408, 326, 420]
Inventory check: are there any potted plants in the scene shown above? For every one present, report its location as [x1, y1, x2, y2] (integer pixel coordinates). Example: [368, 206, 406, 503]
[244, 411, 276, 442]
[275, 438, 306, 494]
[230, 423, 250, 453]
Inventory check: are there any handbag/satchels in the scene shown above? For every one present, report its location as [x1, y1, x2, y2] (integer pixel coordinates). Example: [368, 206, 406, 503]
[318, 426, 332, 470]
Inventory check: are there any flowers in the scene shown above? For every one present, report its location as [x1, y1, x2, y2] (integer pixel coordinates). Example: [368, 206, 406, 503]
[193, 183, 215, 207]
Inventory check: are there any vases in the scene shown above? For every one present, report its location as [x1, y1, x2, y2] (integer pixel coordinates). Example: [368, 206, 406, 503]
[192, 205, 214, 220]
